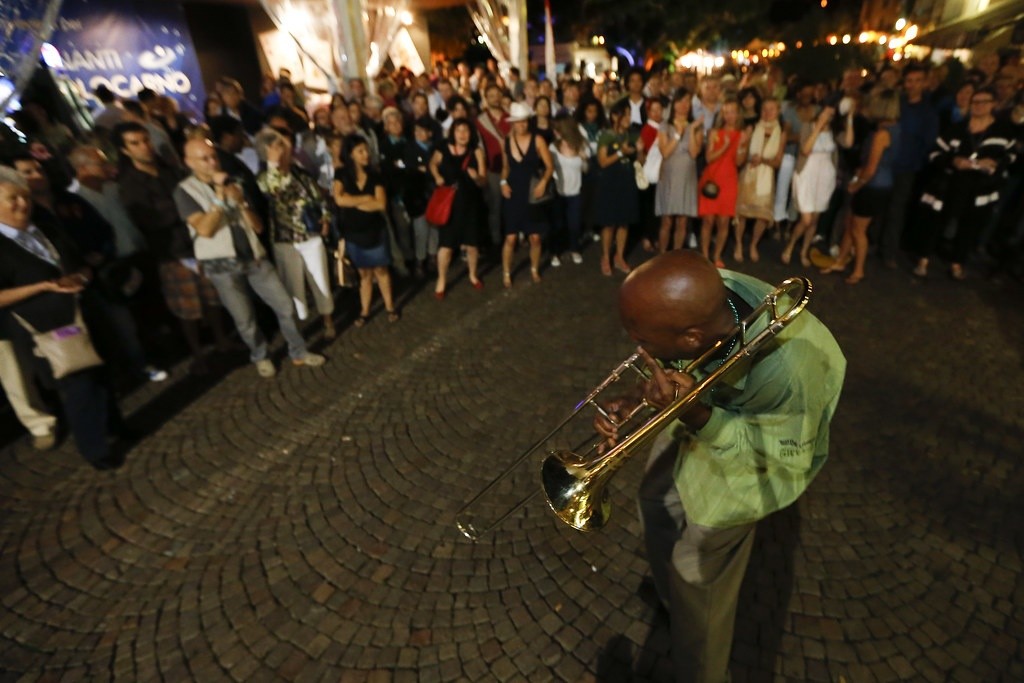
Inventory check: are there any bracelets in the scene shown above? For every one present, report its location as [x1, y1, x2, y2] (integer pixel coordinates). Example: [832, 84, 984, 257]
[500, 179, 507, 185]
[860, 177, 870, 184]
[674, 132, 680, 141]
[970, 159, 979, 170]
[209, 197, 248, 215]
[615, 149, 624, 158]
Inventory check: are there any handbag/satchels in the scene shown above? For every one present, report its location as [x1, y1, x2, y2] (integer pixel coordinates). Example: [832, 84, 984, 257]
[528, 170, 556, 211]
[333, 238, 357, 289]
[32, 322, 106, 379]
[303, 206, 322, 232]
[631, 159, 650, 191]
[424, 185, 457, 226]
[701, 181, 719, 197]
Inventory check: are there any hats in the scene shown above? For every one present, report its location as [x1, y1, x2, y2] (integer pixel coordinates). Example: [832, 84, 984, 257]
[505, 101, 537, 122]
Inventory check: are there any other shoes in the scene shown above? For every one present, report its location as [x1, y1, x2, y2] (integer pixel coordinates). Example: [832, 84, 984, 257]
[322, 324, 336, 342]
[144, 364, 169, 383]
[354, 314, 371, 327]
[255, 356, 278, 378]
[385, 308, 399, 323]
[637, 579, 665, 611]
[92, 456, 125, 472]
[290, 352, 326, 367]
[434, 237, 971, 301]
[119, 427, 145, 442]
[33, 427, 58, 450]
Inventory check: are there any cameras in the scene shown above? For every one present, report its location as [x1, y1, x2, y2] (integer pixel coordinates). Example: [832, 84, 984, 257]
[224, 177, 237, 186]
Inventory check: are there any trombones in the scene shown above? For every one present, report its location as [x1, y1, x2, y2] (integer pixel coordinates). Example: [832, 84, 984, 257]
[454, 274, 815, 541]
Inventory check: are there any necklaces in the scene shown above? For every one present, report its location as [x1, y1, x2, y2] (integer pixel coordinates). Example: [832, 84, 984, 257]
[675, 293, 741, 403]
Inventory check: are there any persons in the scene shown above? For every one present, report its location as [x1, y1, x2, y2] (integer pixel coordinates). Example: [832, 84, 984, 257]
[0, 52, 1024, 470]
[615, 247, 849, 683]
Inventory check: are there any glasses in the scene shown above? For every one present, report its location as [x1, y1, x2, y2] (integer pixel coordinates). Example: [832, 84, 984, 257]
[969, 99, 995, 108]
